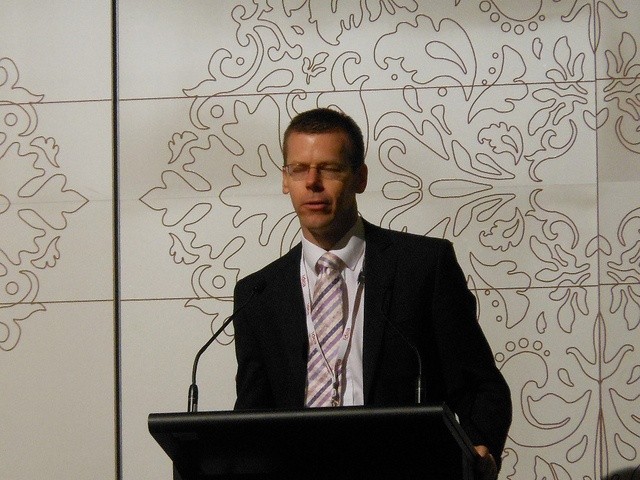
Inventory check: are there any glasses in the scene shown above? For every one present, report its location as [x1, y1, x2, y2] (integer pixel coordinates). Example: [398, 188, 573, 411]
[283, 160, 347, 180]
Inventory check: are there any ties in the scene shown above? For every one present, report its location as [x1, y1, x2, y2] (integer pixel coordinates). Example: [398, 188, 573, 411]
[304, 252, 348, 408]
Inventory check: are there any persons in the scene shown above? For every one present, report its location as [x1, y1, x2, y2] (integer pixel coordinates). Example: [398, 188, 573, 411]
[232, 108, 512, 472]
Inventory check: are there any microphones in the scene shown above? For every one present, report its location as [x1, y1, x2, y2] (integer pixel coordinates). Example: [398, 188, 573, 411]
[187, 280, 268, 413]
